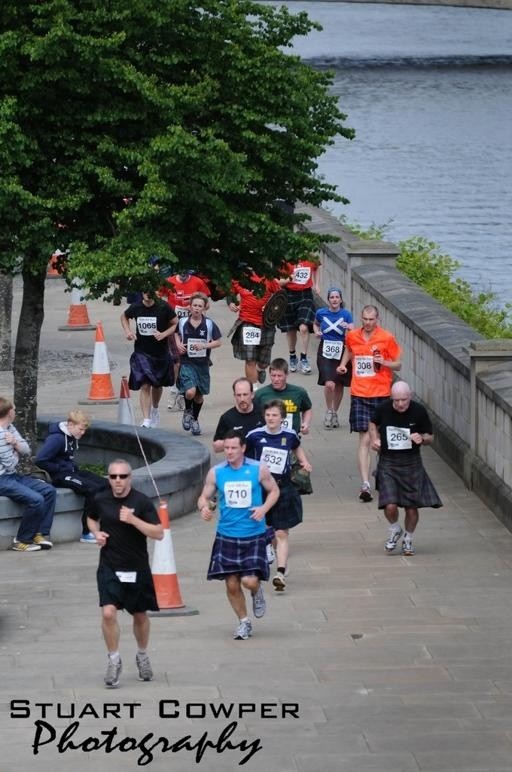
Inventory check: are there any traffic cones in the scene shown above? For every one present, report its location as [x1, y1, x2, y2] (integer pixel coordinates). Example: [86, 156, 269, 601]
[45, 248, 67, 277]
[54, 271, 98, 333]
[74, 320, 124, 406]
[144, 499, 200, 617]
[112, 373, 138, 430]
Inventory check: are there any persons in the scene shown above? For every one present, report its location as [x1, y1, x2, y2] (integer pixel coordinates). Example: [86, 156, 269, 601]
[335, 304, 402, 502]
[35, 410, 110, 544]
[367, 380, 444, 558]
[246, 399, 313, 591]
[313, 286, 355, 429]
[197, 429, 281, 640]
[120, 255, 322, 464]
[86, 458, 166, 686]
[213, 378, 277, 565]
[0, 396, 57, 550]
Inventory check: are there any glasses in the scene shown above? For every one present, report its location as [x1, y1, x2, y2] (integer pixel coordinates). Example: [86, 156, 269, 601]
[110, 474, 128, 479]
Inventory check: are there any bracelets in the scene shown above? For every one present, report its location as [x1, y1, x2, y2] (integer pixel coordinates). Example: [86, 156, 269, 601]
[199, 505, 204, 513]
[13, 439, 17, 446]
[421, 434, 424, 443]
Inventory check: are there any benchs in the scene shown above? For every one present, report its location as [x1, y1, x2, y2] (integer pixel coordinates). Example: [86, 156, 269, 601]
[0, 415, 211, 551]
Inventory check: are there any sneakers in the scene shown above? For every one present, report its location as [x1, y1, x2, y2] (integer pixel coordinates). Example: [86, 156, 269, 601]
[105, 658, 122, 686]
[80, 532, 96, 543]
[182, 409, 200, 434]
[266, 544, 275, 564]
[402, 533, 415, 555]
[385, 527, 403, 551]
[325, 411, 339, 427]
[233, 621, 252, 640]
[136, 655, 153, 680]
[359, 483, 373, 502]
[141, 407, 159, 427]
[272, 573, 285, 591]
[253, 587, 266, 617]
[12, 533, 53, 551]
[290, 357, 311, 373]
[168, 392, 184, 409]
[257, 370, 266, 383]
